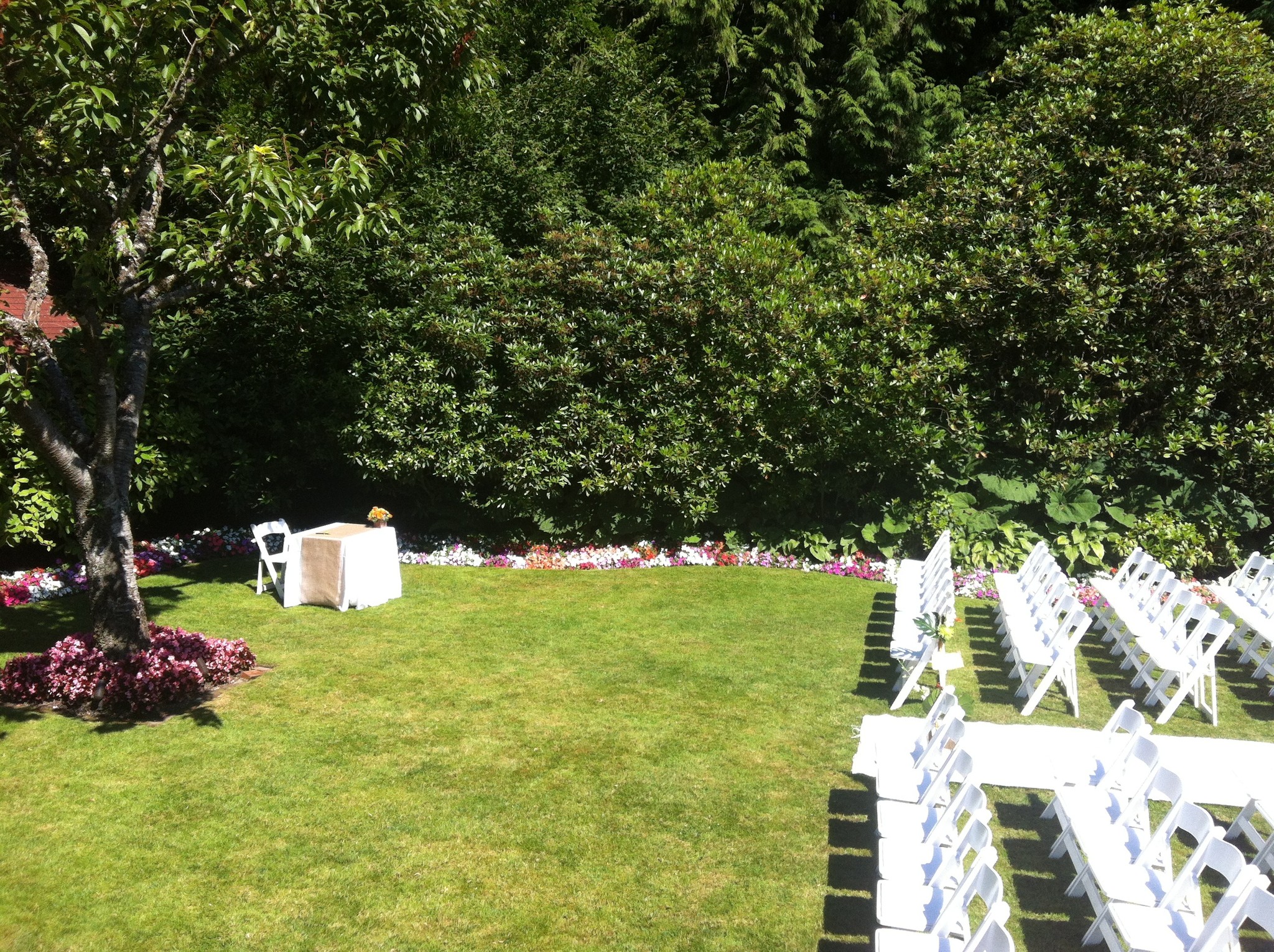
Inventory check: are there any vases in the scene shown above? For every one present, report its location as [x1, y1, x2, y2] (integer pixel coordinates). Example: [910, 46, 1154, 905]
[371, 517, 387, 527]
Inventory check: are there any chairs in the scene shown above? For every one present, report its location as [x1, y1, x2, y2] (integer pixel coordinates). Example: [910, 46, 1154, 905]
[868, 527, 1274, 952]
[250, 517, 291, 600]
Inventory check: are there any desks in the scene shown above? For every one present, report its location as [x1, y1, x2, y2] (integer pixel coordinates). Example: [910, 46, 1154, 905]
[284, 521, 403, 610]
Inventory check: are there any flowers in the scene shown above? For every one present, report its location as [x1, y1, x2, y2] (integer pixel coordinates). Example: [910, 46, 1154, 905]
[363, 505, 393, 521]
[912, 611, 955, 644]
